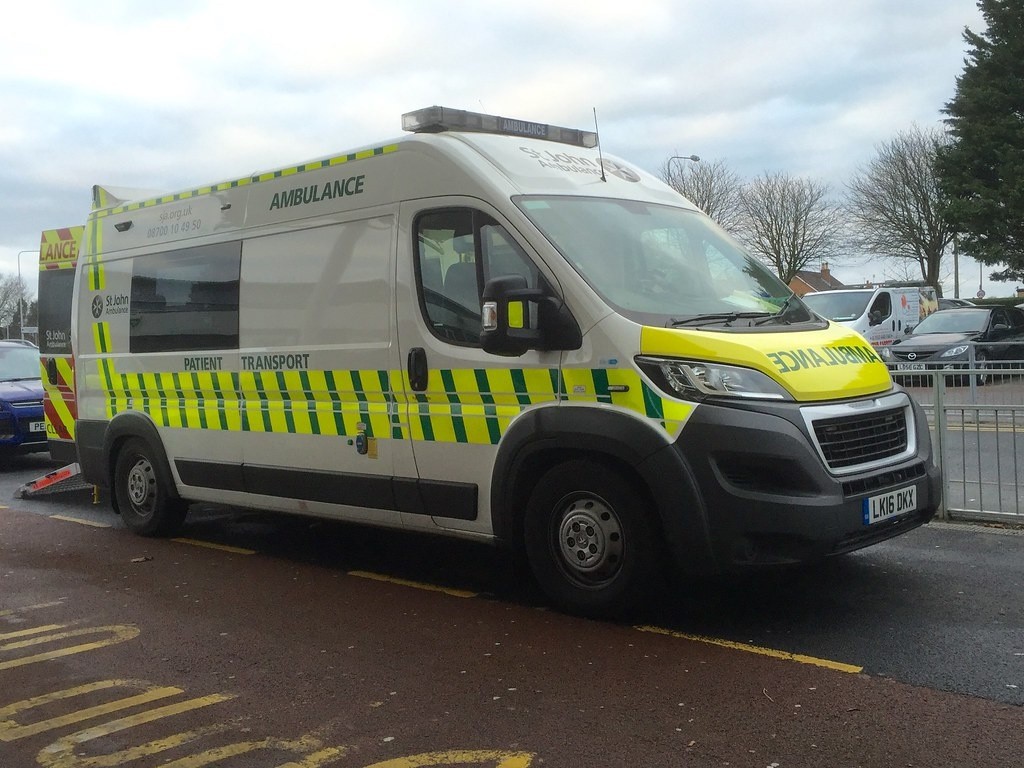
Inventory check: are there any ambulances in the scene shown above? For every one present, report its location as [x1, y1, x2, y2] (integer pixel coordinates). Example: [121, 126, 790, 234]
[16, 107, 944, 619]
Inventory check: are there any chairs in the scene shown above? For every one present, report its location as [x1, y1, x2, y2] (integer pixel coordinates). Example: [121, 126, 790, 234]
[441, 228, 500, 315]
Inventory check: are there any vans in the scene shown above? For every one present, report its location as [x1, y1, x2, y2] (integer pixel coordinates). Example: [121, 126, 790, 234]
[800, 286, 936, 356]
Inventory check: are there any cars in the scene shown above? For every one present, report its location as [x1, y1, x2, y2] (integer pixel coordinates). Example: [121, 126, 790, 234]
[0, 339, 49, 454]
[938, 297, 977, 310]
[880, 305, 1024, 387]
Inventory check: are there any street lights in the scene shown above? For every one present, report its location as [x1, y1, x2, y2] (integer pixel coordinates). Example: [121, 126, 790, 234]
[667, 155, 701, 187]
